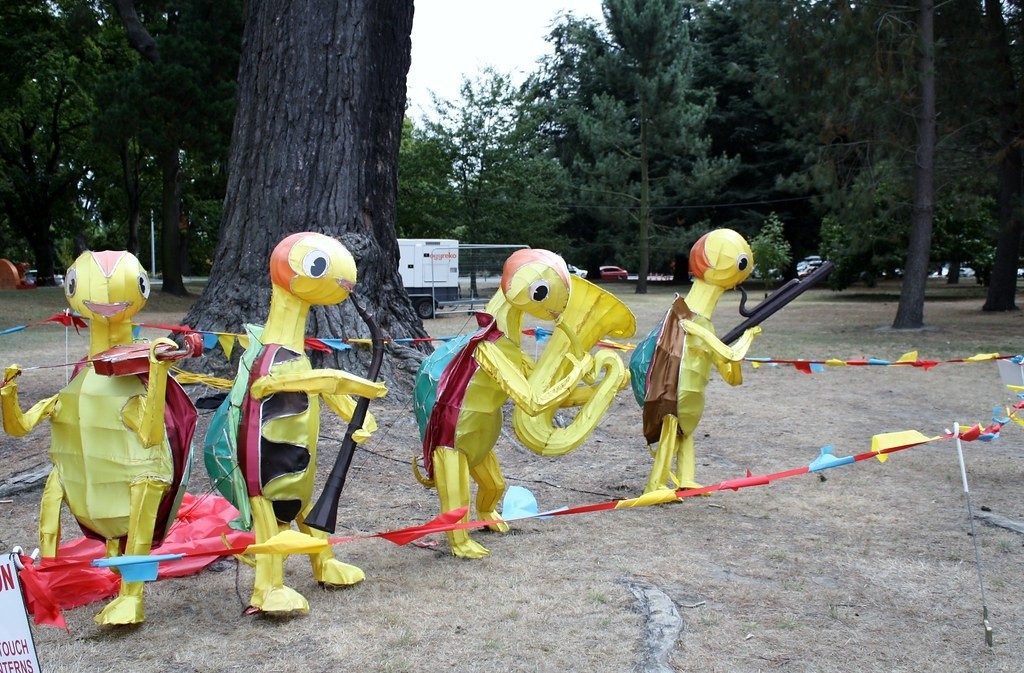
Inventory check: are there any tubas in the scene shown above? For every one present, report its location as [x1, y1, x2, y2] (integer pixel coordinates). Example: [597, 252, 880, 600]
[511, 273, 637, 457]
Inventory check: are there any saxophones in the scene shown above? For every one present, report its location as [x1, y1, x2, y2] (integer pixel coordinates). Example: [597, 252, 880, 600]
[300, 294, 383, 534]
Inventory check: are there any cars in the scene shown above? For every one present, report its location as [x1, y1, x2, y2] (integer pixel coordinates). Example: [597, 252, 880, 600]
[567, 264, 588, 279]
[1017, 267, 1024, 278]
[751, 255, 822, 281]
[929, 261, 976, 279]
[24, 268, 64, 286]
[599, 265, 628, 281]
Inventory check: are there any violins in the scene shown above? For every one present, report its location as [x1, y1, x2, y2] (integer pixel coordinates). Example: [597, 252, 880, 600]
[91, 333, 204, 378]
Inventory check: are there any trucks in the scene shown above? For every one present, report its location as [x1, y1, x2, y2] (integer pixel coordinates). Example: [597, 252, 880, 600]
[397, 238, 460, 319]
